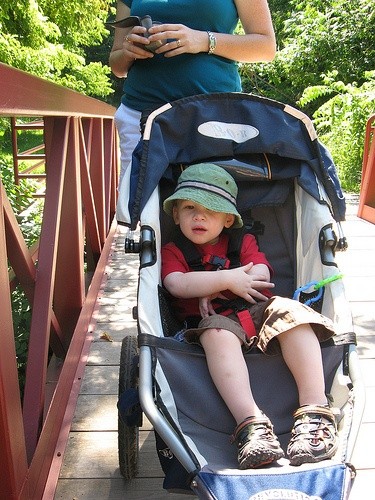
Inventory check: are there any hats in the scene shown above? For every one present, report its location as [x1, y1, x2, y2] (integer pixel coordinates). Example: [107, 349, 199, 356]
[163, 163, 243, 228]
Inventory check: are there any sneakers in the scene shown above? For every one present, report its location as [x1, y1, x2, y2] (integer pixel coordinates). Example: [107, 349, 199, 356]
[286, 402, 340, 466]
[230, 409, 285, 470]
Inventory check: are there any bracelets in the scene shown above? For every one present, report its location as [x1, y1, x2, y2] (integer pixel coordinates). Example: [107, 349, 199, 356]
[207, 30, 216, 55]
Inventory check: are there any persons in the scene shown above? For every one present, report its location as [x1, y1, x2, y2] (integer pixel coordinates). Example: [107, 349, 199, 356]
[107, 0, 276, 190]
[162, 163, 339, 470]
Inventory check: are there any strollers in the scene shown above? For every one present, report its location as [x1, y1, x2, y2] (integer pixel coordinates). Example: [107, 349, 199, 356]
[116, 90, 368, 500]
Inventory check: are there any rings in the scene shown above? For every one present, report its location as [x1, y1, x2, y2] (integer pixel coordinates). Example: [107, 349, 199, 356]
[176, 40, 180, 47]
[125, 36, 131, 42]
